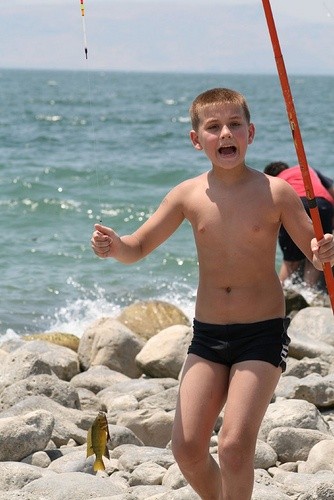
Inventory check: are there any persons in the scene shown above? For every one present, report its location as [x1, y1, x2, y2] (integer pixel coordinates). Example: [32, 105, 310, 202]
[264, 158, 333, 291]
[91, 88, 333, 500]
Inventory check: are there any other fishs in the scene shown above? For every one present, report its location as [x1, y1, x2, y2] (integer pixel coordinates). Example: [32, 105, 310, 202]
[85, 411, 110, 471]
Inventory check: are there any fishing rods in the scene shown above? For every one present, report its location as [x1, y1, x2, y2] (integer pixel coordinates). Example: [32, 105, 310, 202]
[262, 1, 334, 327]
